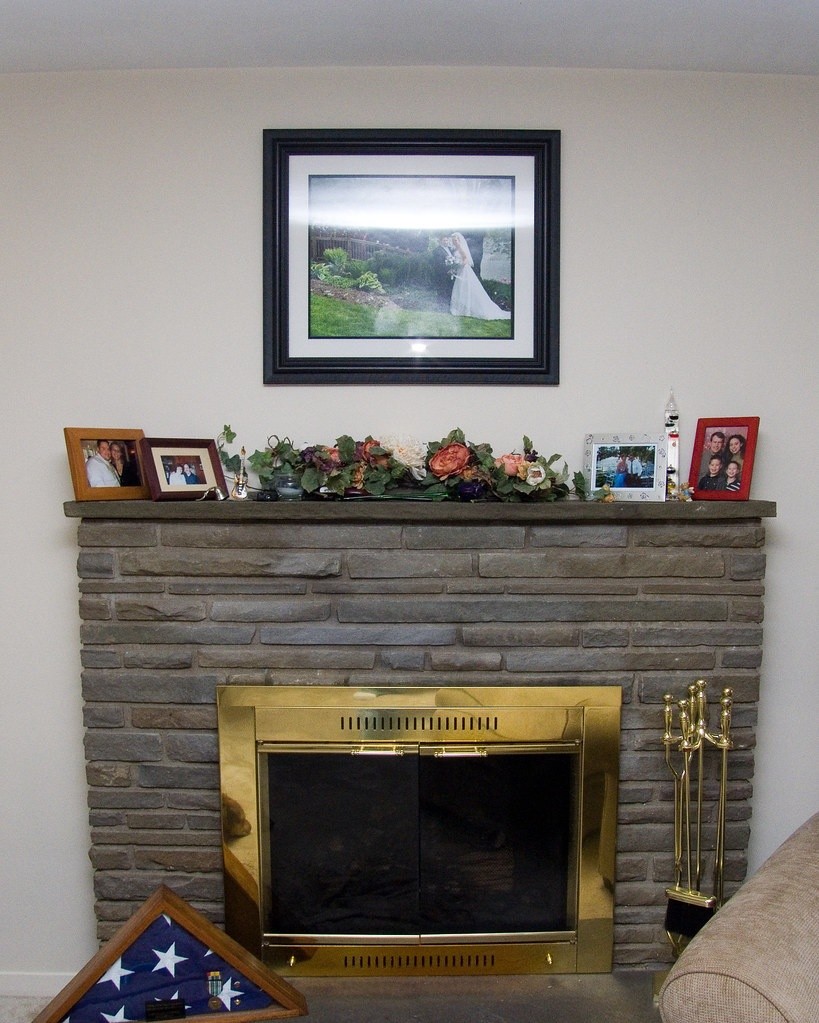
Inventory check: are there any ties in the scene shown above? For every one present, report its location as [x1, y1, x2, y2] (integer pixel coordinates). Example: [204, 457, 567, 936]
[631, 462, 633, 479]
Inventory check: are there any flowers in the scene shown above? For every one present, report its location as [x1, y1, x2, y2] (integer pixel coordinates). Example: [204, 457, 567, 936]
[216, 425, 616, 503]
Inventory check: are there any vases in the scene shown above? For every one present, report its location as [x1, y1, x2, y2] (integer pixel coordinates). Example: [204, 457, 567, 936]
[274, 470, 304, 502]
[454, 481, 485, 501]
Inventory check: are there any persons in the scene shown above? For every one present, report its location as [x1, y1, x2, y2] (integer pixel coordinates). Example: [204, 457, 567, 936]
[86, 440, 141, 490]
[698, 432, 745, 491]
[169, 464, 200, 487]
[614, 454, 643, 488]
[434, 233, 511, 320]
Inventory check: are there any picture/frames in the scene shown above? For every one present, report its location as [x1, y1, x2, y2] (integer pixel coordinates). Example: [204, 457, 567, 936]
[262, 129, 562, 384]
[64, 428, 151, 501]
[688, 416, 760, 500]
[140, 439, 229, 500]
[583, 433, 668, 503]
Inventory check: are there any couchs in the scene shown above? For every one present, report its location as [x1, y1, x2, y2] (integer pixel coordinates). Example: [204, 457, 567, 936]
[658, 811, 819, 1023]
